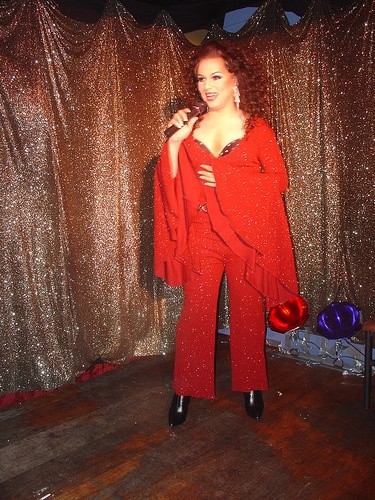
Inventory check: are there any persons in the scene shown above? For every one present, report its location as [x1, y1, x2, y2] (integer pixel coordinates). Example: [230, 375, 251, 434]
[151, 44, 302, 426]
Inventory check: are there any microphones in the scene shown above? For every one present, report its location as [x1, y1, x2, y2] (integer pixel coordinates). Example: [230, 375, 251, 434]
[163, 100, 208, 138]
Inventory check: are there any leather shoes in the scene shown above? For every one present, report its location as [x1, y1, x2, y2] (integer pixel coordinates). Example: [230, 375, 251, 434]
[246, 389, 265, 418]
[168, 393, 190, 427]
